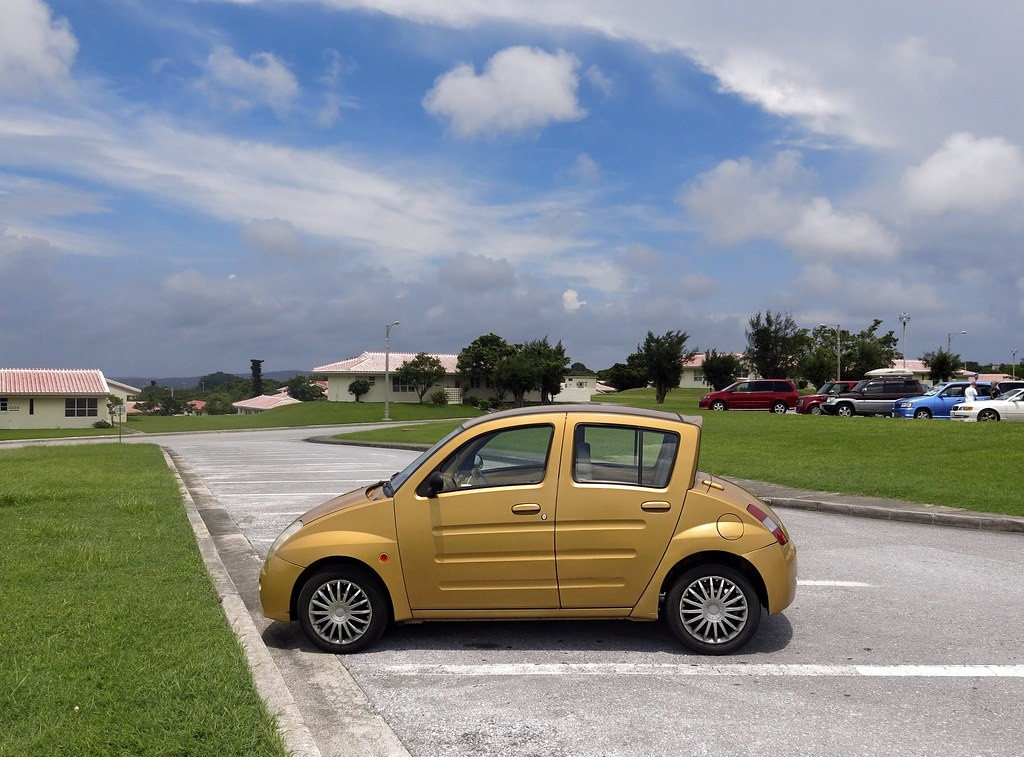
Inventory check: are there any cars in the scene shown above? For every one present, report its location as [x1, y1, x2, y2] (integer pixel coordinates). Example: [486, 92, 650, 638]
[950, 380, 1024, 422]
[259, 404, 798, 656]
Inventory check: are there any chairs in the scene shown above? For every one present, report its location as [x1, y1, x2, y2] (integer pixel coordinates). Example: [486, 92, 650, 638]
[652, 436, 676, 486]
[575, 442, 593, 480]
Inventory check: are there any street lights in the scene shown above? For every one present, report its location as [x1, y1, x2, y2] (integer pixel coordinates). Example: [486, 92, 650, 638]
[820, 323, 841, 381]
[899, 312, 910, 368]
[383, 320, 400, 418]
[948, 330, 966, 354]
[1010, 349, 1017, 380]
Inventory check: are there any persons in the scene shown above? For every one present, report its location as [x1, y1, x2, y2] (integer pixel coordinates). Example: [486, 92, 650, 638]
[989, 381, 1001, 400]
[964, 382, 978, 402]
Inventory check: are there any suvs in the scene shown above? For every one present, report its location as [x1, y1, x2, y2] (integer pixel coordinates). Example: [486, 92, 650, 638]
[795, 380, 861, 415]
[699, 378, 801, 414]
[892, 381, 1002, 420]
[820, 368, 926, 419]
